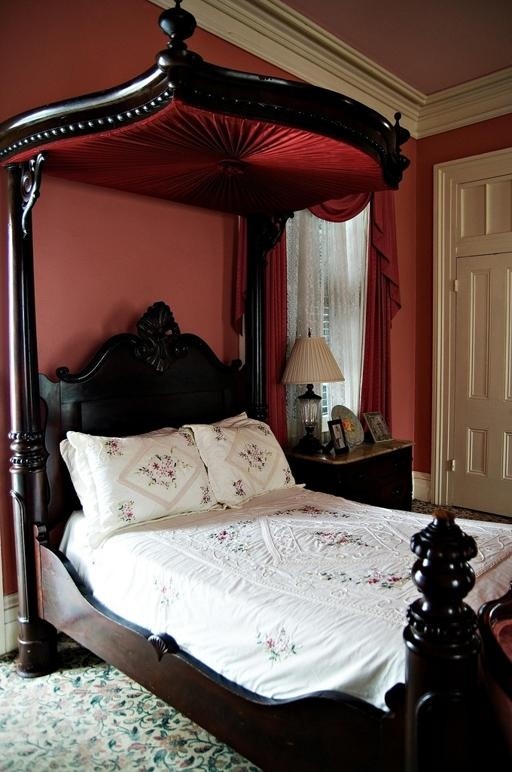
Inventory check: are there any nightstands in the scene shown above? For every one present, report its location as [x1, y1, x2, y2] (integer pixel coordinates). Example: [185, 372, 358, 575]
[288, 435, 416, 513]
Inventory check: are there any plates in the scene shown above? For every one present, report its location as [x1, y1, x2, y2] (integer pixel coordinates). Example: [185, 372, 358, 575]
[331, 405, 364, 445]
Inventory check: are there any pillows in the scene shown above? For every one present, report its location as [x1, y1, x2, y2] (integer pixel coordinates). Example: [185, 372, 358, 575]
[59, 427, 180, 541]
[208, 411, 249, 427]
[66, 427, 229, 551]
[186, 420, 306, 509]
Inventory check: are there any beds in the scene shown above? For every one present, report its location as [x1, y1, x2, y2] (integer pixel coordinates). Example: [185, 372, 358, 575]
[2, 2, 511, 770]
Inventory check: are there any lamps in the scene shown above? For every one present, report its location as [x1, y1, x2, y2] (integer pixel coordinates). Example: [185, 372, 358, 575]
[279, 327, 346, 445]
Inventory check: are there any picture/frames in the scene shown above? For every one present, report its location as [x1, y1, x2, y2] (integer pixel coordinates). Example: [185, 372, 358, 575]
[362, 411, 394, 443]
[327, 419, 350, 457]
